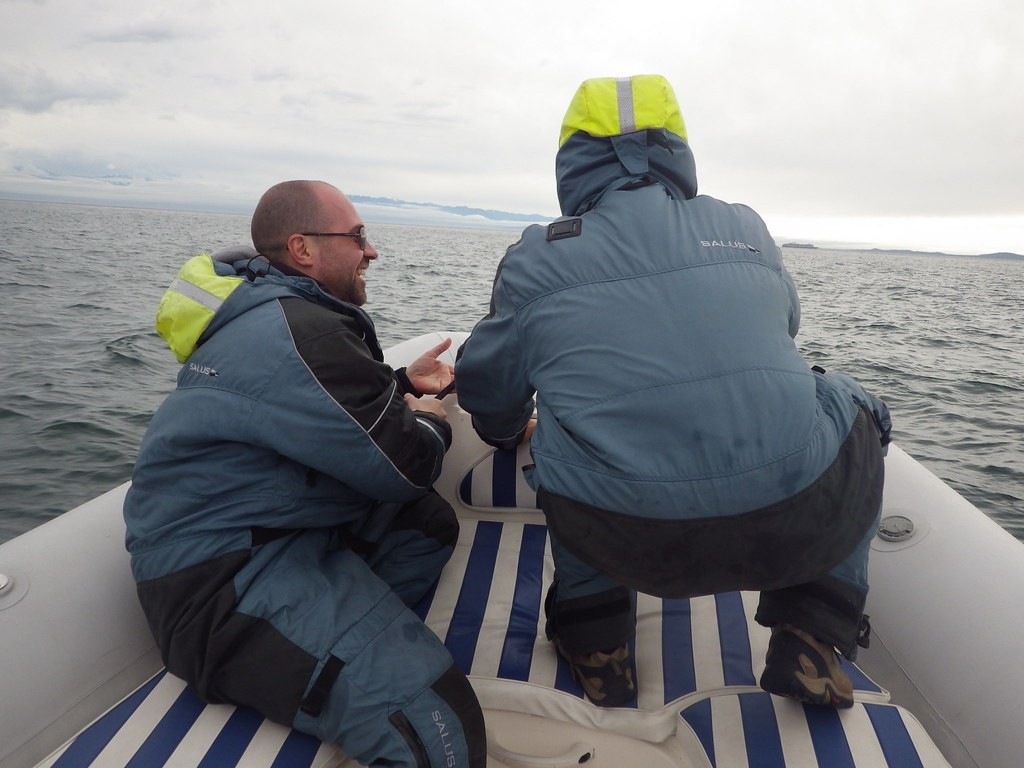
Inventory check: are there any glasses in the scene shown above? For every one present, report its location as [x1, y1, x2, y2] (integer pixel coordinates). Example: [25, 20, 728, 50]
[286, 226, 367, 250]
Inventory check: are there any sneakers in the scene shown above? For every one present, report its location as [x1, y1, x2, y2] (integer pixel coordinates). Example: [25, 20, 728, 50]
[761, 622, 854, 708]
[552, 634, 636, 706]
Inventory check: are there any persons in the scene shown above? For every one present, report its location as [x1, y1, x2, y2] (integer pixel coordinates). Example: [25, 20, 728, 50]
[454, 75, 892, 709]
[124, 180, 489, 768]
